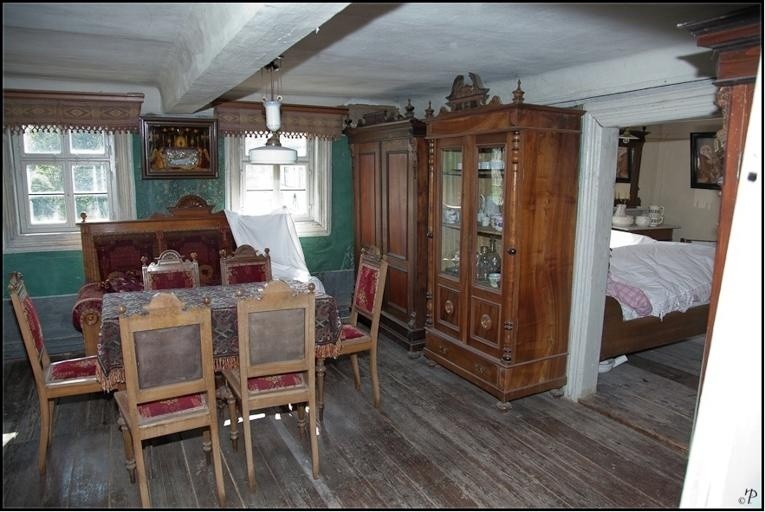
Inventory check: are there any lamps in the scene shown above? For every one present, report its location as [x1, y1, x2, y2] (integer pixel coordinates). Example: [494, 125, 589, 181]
[249, 55, 297, 165]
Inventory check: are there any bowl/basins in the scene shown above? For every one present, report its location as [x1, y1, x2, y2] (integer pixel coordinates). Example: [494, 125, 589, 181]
[636, 216, 651, 226]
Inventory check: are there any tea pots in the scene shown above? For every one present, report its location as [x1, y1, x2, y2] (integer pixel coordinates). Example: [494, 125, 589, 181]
[480, 193, 503, 217]
[648, 205, 665, 219]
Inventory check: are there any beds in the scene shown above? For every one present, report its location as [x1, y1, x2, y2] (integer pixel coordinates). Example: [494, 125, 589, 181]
[599, 230, 717, 362]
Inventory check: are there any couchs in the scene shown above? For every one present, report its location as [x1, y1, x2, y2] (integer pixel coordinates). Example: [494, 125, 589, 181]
[75, 195, 321, 356]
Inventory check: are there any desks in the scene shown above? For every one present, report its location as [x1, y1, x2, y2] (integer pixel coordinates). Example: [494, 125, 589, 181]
[95, 279, 341, 393]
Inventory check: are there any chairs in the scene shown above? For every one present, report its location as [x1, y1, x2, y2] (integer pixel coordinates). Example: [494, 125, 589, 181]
[222, 280, 319, 493]
[113, 293, 226, 508]
[141, 249, 201, 290]
[219, 244, 272, 285]
[6, 271, 116, 476]
[316, 246, 390, 419]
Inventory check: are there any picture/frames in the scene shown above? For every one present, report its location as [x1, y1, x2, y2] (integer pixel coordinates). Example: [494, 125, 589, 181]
[138, 116, 219, 180]
[616, 146, 632, 183]
[690, 131, 725, 189]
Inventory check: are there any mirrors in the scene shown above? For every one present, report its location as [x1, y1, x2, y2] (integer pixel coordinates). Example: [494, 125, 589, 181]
[614, 126, 651, 208]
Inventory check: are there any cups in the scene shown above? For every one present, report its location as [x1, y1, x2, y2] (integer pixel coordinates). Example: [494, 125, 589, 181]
[488, 273, 501, 289]
[478, 160, 505, 169]
[491, 214, 503, 232]
[456, 162, 462, 169]
[649, 217, 664, 227]
[481, 217, 489, 226]
[477, 213, 485, 222]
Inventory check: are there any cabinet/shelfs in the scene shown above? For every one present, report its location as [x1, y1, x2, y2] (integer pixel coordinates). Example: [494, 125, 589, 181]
[341, 98, 428, 360]
[422, 73, 587, 411]
